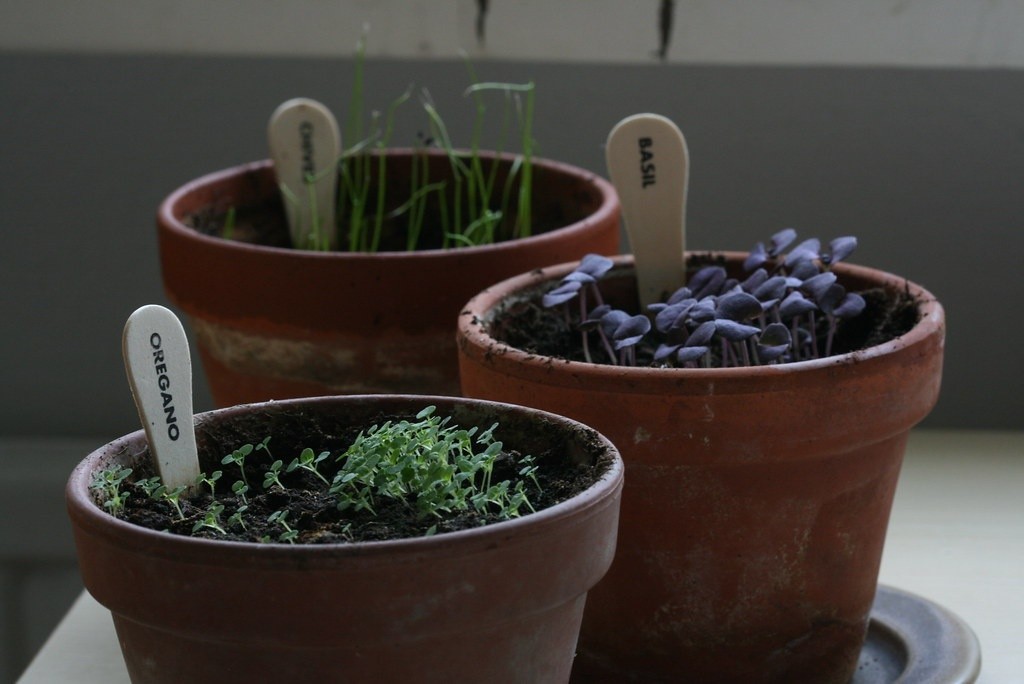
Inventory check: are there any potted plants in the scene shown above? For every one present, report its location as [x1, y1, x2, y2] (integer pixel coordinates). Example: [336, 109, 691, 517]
[157, 15, 622, 417]
[68, 305, 624, 684]
[456, 111, 944, 684]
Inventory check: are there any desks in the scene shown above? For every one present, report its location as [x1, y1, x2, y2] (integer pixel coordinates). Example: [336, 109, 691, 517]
[12, 420, 1024, 684]
